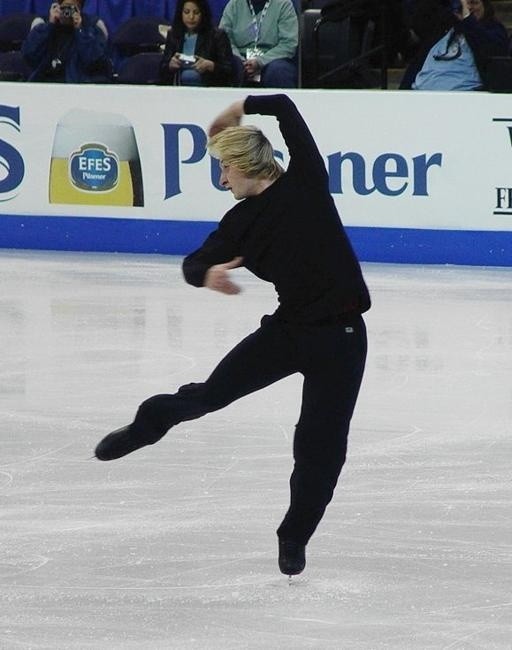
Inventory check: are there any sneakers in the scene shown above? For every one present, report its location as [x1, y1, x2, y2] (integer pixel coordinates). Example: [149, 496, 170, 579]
[278, 536, 306, 574]
[94, 423, 147, 460]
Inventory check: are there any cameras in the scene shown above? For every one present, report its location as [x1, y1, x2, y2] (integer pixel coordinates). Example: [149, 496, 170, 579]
[179, 54, 196, 65]
[451, 0, 461, 12]
[54, 5, 77, 18]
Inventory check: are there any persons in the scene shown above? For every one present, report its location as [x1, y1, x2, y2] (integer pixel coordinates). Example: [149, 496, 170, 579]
[395, 0, 511, 91]
[92, 93, 373, 575]
[218, 0, 298, 87]
[159, 0, 235, 86]
[20, 0, 116, 83]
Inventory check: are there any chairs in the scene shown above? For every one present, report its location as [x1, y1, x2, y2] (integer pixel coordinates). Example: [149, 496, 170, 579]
[0, 0, 247, 84]
